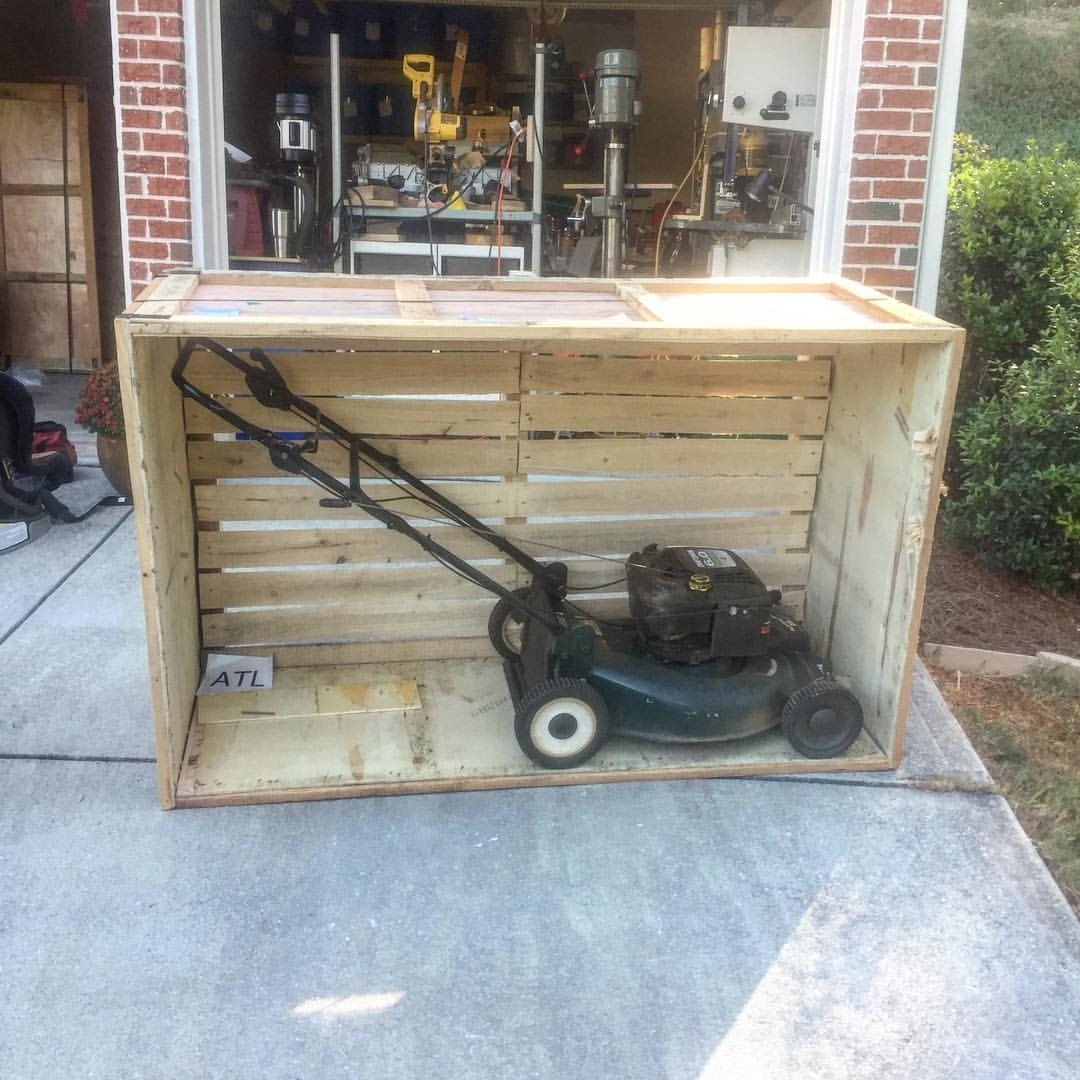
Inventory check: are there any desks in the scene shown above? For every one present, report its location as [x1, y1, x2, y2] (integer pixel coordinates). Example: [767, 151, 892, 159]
[345, 144, 533, 221]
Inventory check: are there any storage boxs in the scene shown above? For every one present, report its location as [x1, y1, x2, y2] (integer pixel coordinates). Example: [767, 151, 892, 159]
[113, 270, 967, 812]
[463, 104, 510, 142]
[346, 185, 400, 202]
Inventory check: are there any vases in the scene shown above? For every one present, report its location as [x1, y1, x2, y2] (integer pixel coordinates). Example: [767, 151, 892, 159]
[96, 433, 132, 499]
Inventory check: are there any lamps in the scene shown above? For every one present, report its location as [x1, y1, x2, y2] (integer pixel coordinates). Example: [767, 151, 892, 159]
[744, 168, 815, 216]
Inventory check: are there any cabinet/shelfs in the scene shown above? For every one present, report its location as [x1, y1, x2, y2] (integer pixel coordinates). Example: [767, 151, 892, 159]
[708, 24, 829, 277]
[1, 81, 120, 372]
[496, 74, 630, 184]
[282, 55, 492, 145]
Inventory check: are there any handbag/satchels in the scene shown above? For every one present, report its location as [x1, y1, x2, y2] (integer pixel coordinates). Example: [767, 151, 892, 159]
[34, 421, 77, 466]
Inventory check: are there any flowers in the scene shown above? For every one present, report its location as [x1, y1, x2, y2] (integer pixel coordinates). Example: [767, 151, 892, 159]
[74, 362, 126, 435]
